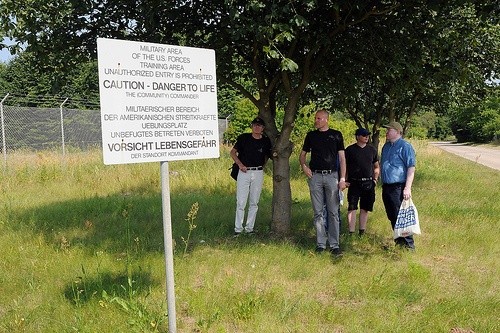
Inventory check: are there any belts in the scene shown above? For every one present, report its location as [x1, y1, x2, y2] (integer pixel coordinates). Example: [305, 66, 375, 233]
[311, 170, 337, 176]
[352, 177, 372, 181]
[247, 167, 262, 170]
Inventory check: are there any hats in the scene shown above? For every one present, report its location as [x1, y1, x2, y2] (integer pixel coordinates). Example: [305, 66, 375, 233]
[354, 128, 370, 137]
[251, 117, 264, 125]
[382, 121, 403, 133]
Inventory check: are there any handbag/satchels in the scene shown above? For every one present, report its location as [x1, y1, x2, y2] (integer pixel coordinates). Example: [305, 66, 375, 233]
[393, 197, 421, 239]
[228, 163, 239, 181]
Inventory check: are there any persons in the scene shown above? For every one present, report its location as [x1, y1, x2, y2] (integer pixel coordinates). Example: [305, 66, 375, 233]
[299, 110, 346, 254]
[345, 128, 380, 237]
[229, 118, 273, 238]
[380, 122, 416, 253]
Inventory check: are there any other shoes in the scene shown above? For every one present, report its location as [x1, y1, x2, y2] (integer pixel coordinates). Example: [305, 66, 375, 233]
[330, 246, 342, 255]
[315, 246, 326, 252]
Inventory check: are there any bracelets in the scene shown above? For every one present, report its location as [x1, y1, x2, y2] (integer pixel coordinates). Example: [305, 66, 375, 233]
[340, 178, 346, 183]
[373, 177, 378, 184]
